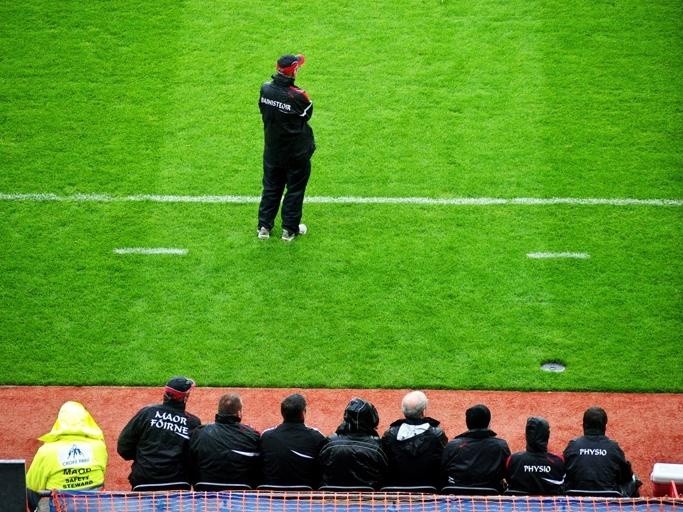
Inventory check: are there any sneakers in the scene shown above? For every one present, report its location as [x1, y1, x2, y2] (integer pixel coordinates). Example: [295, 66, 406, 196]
[256, 227, 270, 240]
[280, 224, 307, 241]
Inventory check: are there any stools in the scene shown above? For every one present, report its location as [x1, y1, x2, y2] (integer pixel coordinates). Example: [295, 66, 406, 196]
[648, 459, 683, 498]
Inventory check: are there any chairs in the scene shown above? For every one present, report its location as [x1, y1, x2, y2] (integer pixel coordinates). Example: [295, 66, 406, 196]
[30, 479, 626, 510]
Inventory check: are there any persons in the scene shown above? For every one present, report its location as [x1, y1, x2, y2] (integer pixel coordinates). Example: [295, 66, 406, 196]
[504, 417, 568, 494]
[255, 55, 315, 243]
[26, 400, 109, 511]
[182, 393, 260, 490]
[560, 404, 642, 496]
[258, 394, 326, 486]
[319, 396, 388, 490]
[116, 378, 201, 491]
[380, 389, 447, 486]
[441, 403, 510, 490]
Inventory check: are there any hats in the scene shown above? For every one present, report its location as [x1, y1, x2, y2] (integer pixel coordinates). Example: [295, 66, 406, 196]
[274, 54, 304, 76]
[163, 377, 195, 399]
